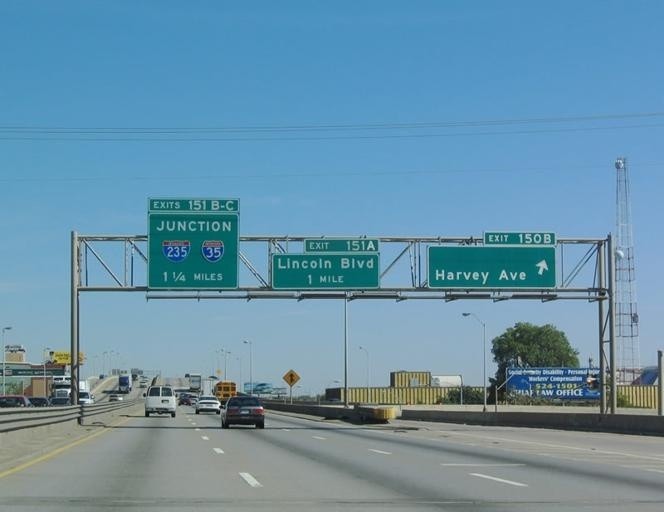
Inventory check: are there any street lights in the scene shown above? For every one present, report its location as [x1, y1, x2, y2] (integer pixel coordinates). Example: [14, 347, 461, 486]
[237, 356, 242, 393]
[2, 325, 12, 396]
[43, 347, 50, 398]
[463, 311, 487, 412]
[243, 338, 253, 394]
[360, 346, 370, 387]
[220, 346, 231, 380]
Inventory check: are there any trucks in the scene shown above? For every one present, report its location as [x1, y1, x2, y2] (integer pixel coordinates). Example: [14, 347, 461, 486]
[119, 373, 132, 393]
[190, 374, 202, 392]
[52, 376, 88, 405]
[211, 381, 237, 405]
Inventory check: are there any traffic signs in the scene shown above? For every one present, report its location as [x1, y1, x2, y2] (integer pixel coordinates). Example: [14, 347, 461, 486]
[147, 212, 240, 290]
[272, 252, 379, 289]
[427, 246, 556, 289]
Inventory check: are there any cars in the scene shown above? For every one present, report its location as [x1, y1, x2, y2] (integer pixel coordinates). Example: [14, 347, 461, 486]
[179, 393, 199, 406]
[133, 373, 138, 381]
[196, 395, 220, 414]
[143, 384, 178, 417]
[109, 394, 123, 402]
[219, 396, 265, 429]
[0, 395, 72, 406]
[140, 378, 148, 388]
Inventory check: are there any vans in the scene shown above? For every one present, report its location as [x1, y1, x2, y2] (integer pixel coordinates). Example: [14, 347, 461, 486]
[79, 391, 91, 405]
